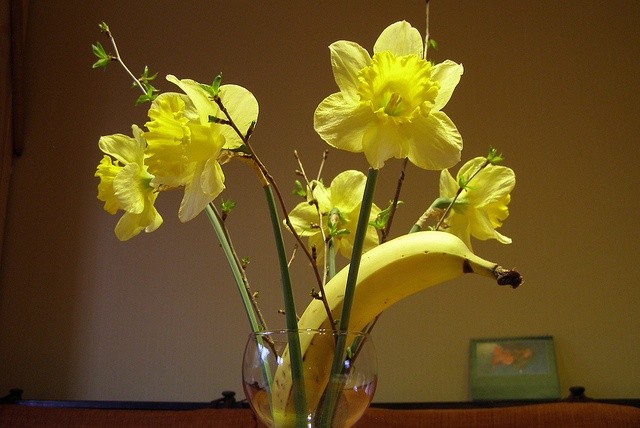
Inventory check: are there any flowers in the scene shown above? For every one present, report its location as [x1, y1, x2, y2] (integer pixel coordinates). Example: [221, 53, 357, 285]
[94, 20, 516, 428]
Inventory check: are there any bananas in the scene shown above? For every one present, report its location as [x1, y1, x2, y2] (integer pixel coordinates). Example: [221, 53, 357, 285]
[271, 230, 524, 428]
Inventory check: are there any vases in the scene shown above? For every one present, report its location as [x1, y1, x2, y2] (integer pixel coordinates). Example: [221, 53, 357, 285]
[241, 328, 378, 428]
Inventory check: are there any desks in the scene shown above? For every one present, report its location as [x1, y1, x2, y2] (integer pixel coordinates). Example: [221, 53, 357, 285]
[0, 401, 640, 428]
